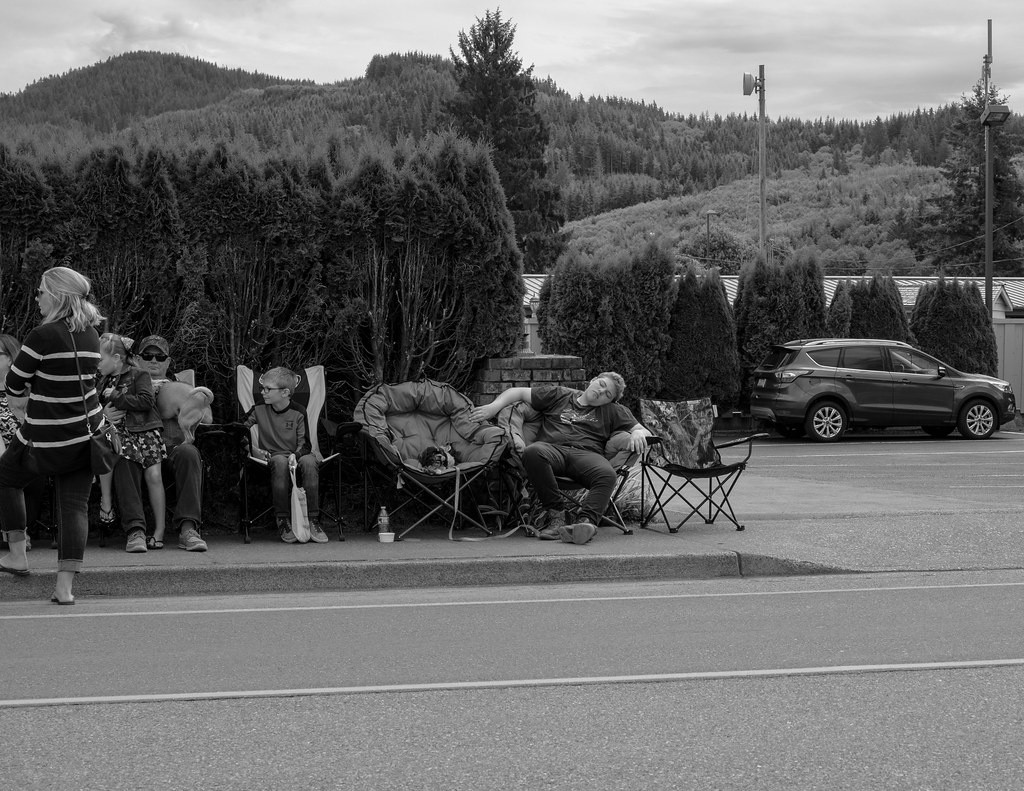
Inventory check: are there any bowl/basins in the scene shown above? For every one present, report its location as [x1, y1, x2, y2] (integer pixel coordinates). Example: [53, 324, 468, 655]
[379, 533, 395, 543]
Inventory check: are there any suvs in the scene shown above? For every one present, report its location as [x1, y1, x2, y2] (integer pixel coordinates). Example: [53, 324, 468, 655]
[750, 338, 1016, 442]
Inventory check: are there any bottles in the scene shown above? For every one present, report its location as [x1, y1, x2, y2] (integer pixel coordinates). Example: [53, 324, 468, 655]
[378, 507, 389, 541]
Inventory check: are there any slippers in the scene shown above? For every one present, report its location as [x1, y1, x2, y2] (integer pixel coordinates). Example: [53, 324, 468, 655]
[146, 533, 164, 550]
[51, 591, 75, 605]
[0, 560, 30, 575]
[99, 496, 115, 524]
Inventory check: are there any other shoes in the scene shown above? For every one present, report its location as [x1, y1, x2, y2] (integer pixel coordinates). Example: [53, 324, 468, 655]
[25, 534, 32, 551]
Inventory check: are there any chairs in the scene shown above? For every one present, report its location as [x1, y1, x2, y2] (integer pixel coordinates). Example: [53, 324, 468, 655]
[236, 364, 771, 544]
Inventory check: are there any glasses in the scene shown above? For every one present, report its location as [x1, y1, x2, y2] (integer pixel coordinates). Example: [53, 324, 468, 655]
[34, 287, 52, 298]
[140, 353, 167, 362]
[260, 386, 285, 393]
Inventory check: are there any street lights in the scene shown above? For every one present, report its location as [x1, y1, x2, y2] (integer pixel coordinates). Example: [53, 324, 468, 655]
[744, 64, 766, 254]
[707, 209, 717, 269]
[979, 19, 1011, 318]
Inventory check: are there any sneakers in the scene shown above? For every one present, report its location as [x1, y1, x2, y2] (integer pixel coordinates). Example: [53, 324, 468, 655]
[276, 518, 297, 543]
[177, 529, 208, 551]
[559, 522, 598, 545]
[308, 518, 328, 543]
[539, 518, 567, 540]
[125, 530, 147, 552]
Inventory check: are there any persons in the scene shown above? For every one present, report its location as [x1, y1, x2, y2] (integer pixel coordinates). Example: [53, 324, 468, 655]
[97, 333, 208, 552]
[0, 267, 103, 605]
[237, 367, 329, 543]
[469, 372, 652, 544]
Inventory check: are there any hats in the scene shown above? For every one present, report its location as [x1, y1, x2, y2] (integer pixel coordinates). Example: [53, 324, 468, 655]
[139, 335, 169, 356]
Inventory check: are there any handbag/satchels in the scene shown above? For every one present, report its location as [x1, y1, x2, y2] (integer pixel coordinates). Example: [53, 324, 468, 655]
[88, 418, 124, 475]
[290, 466, 310, 543]
